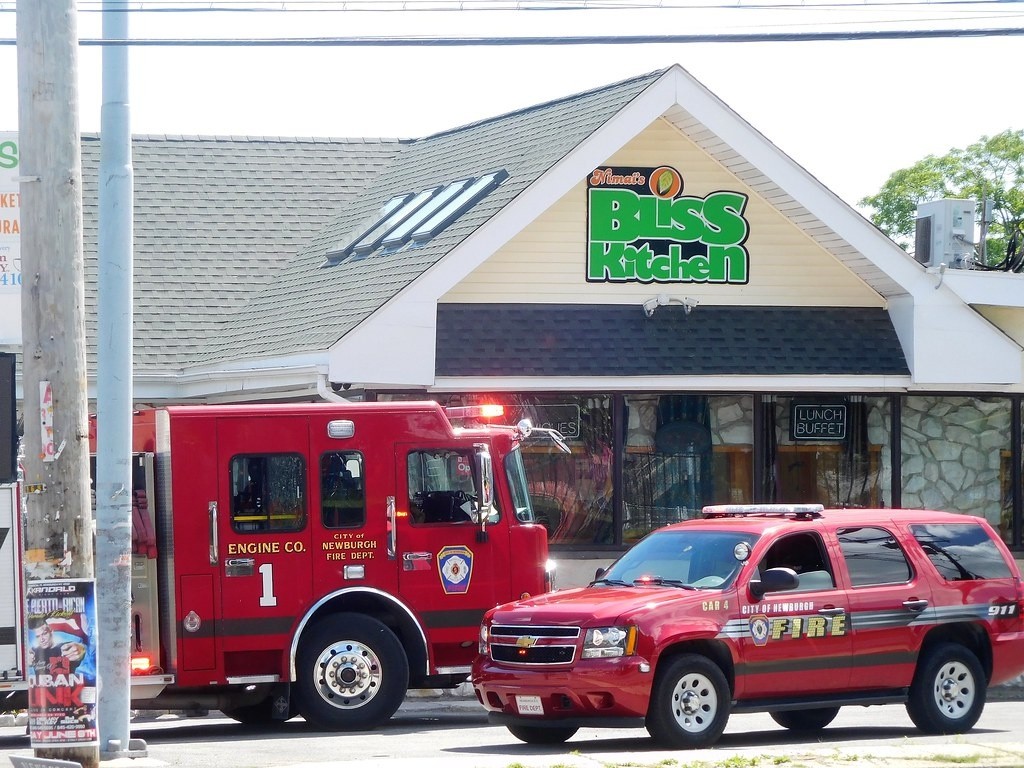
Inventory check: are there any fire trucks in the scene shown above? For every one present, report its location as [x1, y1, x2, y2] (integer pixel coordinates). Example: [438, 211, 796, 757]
[1, 345, 574, 732]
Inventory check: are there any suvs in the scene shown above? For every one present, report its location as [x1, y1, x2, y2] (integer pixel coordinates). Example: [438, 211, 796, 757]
[471, 504, 1024, 751]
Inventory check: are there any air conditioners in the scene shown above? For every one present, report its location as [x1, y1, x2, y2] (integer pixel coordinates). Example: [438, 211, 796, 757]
[914, 198, 975, 269]
